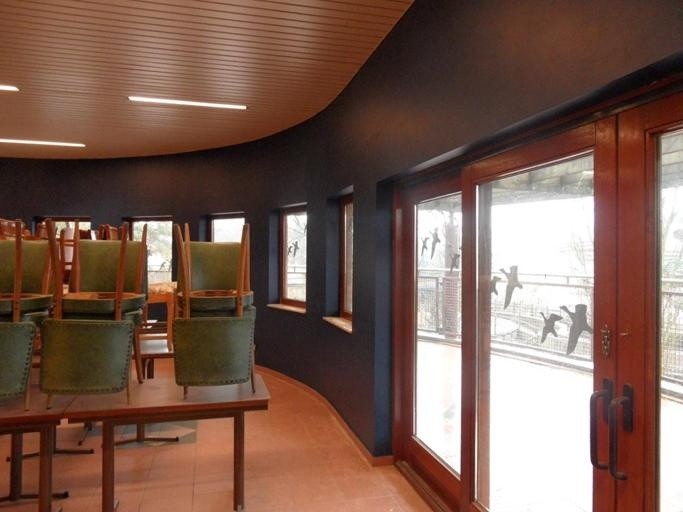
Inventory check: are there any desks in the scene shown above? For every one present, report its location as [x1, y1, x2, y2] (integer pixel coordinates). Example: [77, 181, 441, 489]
[1, 319, 270, 512]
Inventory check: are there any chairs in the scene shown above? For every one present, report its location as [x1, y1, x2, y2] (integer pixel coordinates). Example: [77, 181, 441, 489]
[1, 216, 256, 411]
